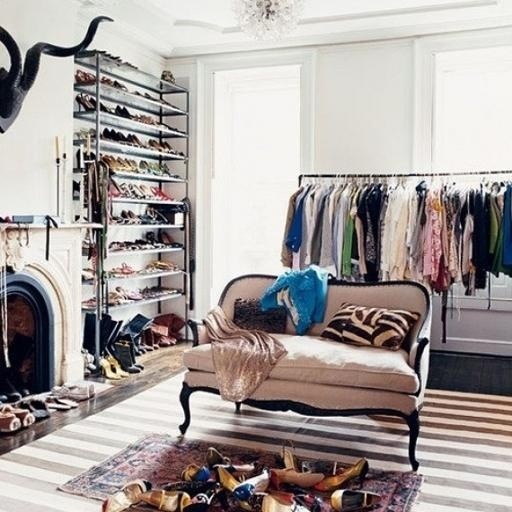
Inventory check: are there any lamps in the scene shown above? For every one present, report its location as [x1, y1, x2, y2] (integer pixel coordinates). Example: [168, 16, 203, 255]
[232, 0, 304, 44]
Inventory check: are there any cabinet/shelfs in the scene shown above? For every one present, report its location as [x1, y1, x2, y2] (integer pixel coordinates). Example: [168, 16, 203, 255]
[72, 52, 190, 376]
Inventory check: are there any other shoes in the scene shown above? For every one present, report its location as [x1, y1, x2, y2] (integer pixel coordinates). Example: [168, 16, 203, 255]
[1, 383, 94, 432]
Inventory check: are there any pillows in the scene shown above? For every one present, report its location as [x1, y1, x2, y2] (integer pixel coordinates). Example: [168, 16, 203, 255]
[232, 297, 288, 334]
[320, 301, 421, 351]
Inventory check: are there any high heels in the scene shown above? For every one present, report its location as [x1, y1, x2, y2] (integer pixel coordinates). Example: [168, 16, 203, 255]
[103, 439, 381, 512]
[74, 51, 184, 379]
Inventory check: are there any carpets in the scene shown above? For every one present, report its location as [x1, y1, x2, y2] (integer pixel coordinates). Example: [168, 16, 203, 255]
[58, 431, 424, 512]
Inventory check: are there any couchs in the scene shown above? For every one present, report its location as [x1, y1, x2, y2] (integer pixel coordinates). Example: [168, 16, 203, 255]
[179, 274, 433, 472]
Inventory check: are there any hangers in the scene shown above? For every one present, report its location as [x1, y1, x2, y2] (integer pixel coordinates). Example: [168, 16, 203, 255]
[299, 170, 512, 197]
